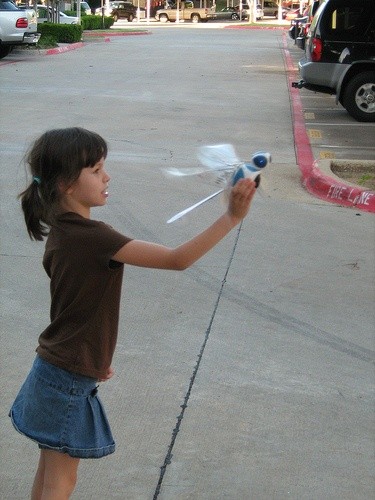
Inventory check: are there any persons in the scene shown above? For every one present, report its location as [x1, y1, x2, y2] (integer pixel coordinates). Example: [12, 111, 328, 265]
[7, 126, 257, 499]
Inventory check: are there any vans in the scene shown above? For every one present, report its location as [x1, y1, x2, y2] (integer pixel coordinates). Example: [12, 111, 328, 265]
[235, 5, 264, 20]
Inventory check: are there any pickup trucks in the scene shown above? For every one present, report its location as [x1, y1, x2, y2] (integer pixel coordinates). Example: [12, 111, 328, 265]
[154, 1, 216, 23]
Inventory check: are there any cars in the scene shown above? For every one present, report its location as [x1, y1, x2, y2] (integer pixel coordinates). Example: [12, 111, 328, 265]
[19, 5, 80, 29]
[216, 6, 247, 20]
[0, 0, 41, 60]
[263, 1, 291, 18]
[288, 0, 323, 49]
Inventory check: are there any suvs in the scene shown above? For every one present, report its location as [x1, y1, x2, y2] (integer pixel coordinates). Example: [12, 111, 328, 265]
[292, 0, 375, 123]
[95, 1, 135, 22]
